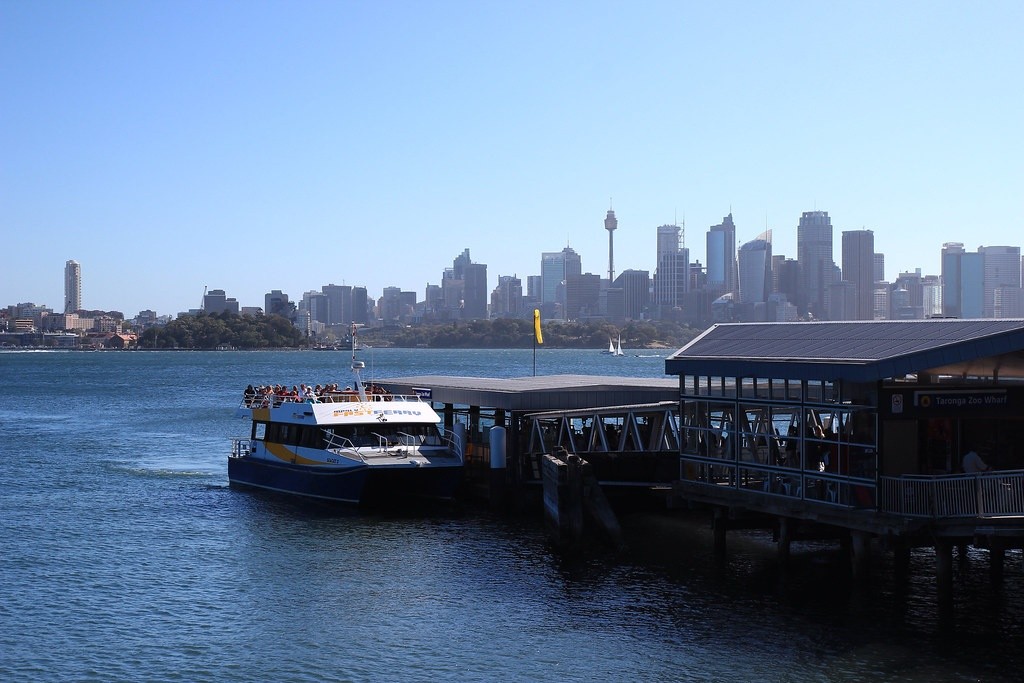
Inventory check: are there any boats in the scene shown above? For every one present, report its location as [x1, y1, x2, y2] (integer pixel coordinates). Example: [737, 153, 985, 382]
[227, 322, 465, 519]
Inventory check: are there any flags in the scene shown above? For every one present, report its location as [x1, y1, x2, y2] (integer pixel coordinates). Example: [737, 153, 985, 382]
[534, 309, 544, 345]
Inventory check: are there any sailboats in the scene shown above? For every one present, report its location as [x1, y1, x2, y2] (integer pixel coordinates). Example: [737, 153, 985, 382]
[601, 336, 614, 354]
[612, 334, 625, 358]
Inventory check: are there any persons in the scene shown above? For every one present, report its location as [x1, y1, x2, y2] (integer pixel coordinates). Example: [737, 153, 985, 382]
[241, 383, 342, 408]
[786, 425, 849, 471]
[372, 385, 393, 401]
[345, 385, 351, 401]
